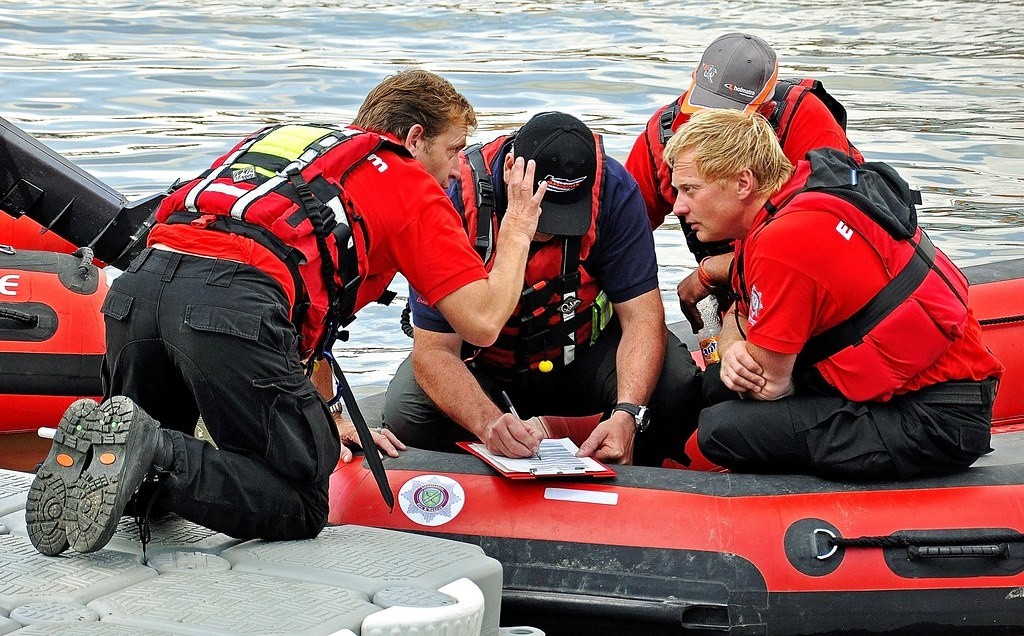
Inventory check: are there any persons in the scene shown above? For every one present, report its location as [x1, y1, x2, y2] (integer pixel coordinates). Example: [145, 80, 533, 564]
[24, 69, 548, 562]
[383, 111, 701, 467]
[662, 107, 1005, 482]
[321, 400, 343, 415]
[620, 31, 865, 304]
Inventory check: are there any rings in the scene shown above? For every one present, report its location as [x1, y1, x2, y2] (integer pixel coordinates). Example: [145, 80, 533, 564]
[377, 425, 381, 434]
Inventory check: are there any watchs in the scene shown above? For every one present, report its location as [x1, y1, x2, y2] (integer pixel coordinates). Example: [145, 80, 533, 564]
[610, 403, 651, 433]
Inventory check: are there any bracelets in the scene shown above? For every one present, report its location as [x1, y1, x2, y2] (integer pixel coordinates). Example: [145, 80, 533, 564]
[698, 255, 721, 291]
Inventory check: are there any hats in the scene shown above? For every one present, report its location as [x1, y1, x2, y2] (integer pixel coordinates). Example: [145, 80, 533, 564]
[679, 33, 778, 117]
[514, 110, 597, 235]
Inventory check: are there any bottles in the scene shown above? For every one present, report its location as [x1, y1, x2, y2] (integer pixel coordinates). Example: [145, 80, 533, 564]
[695, 295, 723, 366]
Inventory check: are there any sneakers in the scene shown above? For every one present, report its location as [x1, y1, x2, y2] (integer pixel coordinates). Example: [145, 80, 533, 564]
[23, 395, 160, 558]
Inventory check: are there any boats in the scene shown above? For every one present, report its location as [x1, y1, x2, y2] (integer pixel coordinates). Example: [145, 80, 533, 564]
[1, 118, 178, 434]
[323, 259, 1024, 636]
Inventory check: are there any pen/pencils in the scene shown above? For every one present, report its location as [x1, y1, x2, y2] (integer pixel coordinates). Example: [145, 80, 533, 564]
[502, 390, 544, 461]
